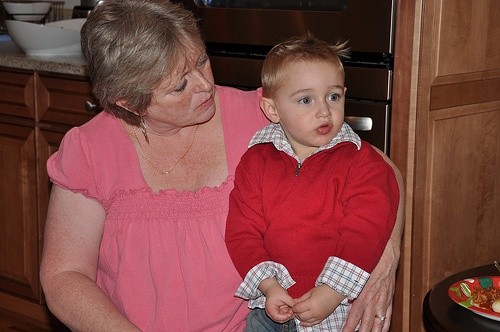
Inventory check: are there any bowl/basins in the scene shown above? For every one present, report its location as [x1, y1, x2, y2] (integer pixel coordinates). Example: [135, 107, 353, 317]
[2, 2, 52, 21]
[5, 20, 80, 50]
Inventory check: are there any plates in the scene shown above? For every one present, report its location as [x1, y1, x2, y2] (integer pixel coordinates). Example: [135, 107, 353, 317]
[25, 18, 87, 65]
[448, 276, 500, 321]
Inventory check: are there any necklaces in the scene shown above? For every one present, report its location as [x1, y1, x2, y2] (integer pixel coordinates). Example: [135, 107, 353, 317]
[131, 123, 199, 175]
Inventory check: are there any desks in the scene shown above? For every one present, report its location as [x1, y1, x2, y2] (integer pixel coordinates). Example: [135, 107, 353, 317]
[422, 259, 500, 332]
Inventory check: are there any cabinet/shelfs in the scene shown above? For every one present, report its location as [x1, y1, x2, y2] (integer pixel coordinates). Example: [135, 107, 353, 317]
[0, 0, 395, 332]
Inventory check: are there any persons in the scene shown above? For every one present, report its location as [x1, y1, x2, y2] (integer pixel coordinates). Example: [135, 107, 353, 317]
[39, 0, 404, 332]
[225, 35, 400, 332]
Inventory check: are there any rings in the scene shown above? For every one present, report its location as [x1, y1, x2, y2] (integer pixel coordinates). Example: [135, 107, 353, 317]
[375, 315, 386, 321]
